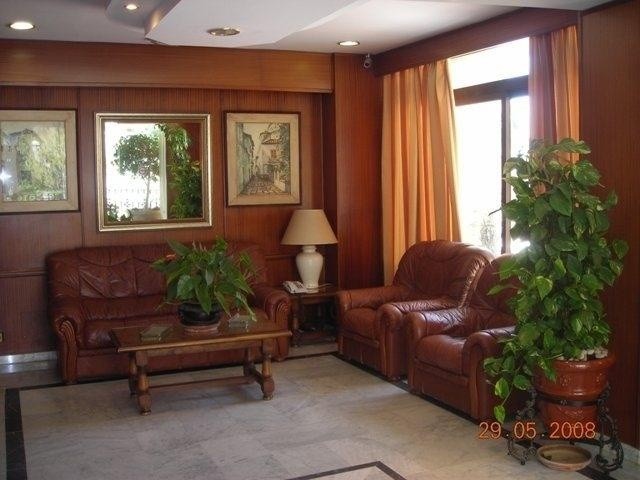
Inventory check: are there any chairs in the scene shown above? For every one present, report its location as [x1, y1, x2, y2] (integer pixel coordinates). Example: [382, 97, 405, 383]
[333, 240, 535, 426]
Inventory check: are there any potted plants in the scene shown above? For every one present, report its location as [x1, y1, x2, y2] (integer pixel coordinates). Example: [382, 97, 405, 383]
[148, 231, 260, 335]
[482, 134, 630, 438]
[110, 133, 161, 223]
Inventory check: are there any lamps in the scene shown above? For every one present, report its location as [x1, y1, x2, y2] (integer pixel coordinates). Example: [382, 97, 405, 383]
[279, 210, 340, 289]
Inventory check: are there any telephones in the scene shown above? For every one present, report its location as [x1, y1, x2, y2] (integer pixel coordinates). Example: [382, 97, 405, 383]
[283, 281, 307, 294]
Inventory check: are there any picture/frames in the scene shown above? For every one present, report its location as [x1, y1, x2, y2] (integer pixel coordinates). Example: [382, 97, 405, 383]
[0, 109, 80, 215]
[221, 111, 303, 208]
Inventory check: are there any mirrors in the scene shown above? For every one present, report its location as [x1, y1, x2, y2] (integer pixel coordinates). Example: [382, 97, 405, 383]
[94, 112, 213, 232]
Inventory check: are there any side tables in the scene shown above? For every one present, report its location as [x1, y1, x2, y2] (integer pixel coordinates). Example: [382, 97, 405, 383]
[280, 283, 341, 347]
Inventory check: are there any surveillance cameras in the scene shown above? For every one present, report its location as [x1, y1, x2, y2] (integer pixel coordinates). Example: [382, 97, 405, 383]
[364, 53, 373, 68]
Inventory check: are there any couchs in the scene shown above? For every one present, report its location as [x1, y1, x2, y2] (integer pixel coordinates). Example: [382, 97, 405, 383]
[46, 241, 292, 384]
